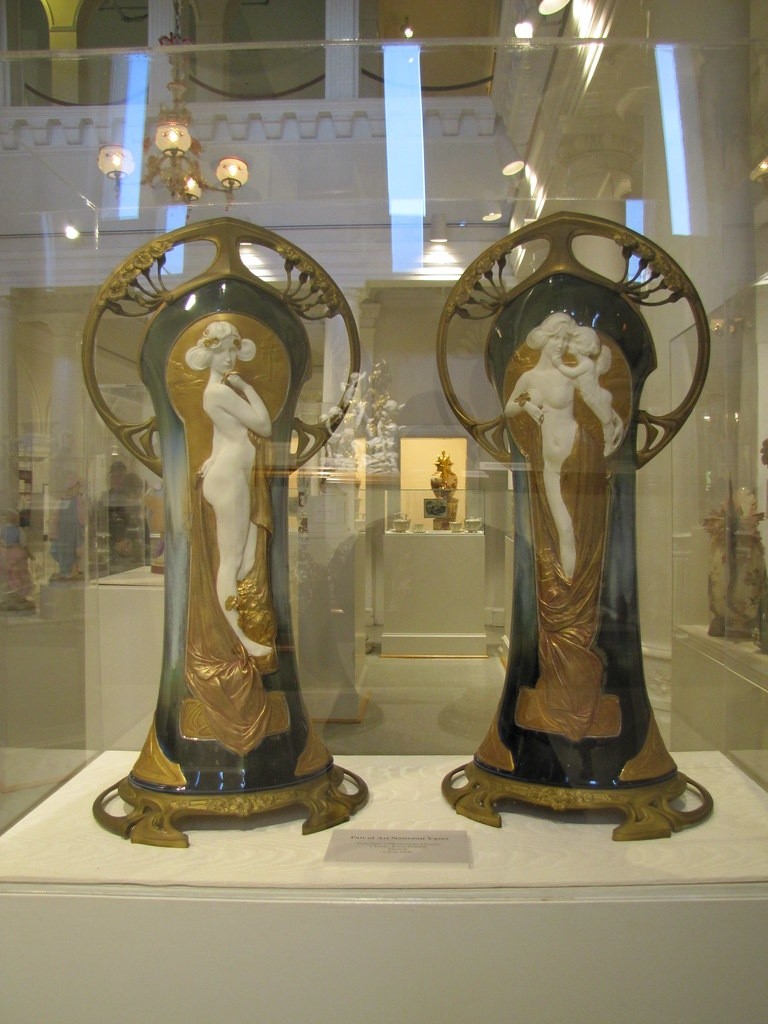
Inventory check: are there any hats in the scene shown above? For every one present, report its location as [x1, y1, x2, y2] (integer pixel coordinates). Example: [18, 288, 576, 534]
[108, 461, 126, 474]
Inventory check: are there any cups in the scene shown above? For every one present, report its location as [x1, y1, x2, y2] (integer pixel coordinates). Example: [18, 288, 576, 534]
[412, 523, 424, 532]
[465, 518, 481, 533]
[393, 519, 410, 533]
[449, 522, 462, 532]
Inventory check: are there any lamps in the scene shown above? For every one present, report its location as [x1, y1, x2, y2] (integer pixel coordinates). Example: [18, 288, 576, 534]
[493, 114, 524, 176]
[513, 6, 543, 39]
[536, 0, 570, 15]
[479, 179, 502, 221]
[97, 32, 249, 205]
[430, 214, 447, 244]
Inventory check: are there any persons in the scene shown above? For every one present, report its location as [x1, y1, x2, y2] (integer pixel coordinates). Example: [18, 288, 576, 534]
[299, 390, 534, 682]
[185, 321, 272, 656]
[0, 461, 165, 601]
[504, 313, 623, 579]
